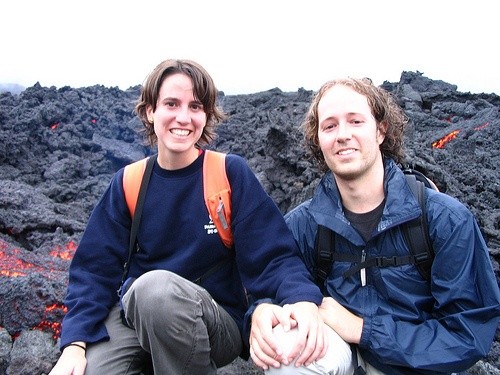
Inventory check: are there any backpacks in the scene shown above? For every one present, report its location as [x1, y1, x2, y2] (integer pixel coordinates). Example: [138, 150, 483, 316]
[311, 162, 442, 295]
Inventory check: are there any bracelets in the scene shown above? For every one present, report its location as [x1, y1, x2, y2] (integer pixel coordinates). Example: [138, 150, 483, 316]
[62, 344, 86, 353]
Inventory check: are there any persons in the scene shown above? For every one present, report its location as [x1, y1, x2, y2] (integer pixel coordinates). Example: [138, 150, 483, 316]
[244, 77, 500, 375]
[46, 58, 324, 375]
